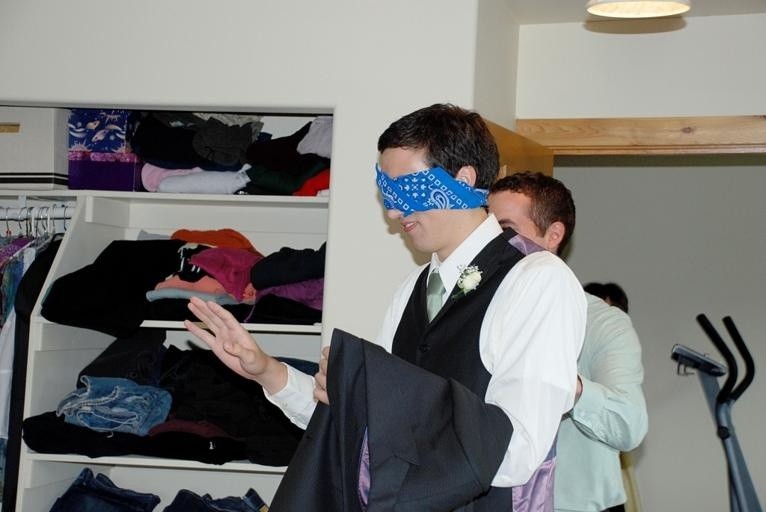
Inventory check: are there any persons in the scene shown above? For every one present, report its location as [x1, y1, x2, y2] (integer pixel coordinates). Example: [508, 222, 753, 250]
[484, 170, 648, 511]
[584, 281, 639, 512]
[183, 103, 587, 511]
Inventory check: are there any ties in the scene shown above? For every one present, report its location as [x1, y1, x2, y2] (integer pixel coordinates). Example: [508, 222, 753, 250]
[426, 271, 446, 327]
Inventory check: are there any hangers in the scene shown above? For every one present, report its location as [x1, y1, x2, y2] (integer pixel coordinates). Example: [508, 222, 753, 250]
[2, 204, 72, 263]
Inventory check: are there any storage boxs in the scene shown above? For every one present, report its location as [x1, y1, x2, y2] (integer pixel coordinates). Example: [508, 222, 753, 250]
[0, 105, 72, 183]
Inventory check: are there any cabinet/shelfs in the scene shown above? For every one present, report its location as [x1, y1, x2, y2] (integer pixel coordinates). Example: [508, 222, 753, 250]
[16, 101, 420, 505]
